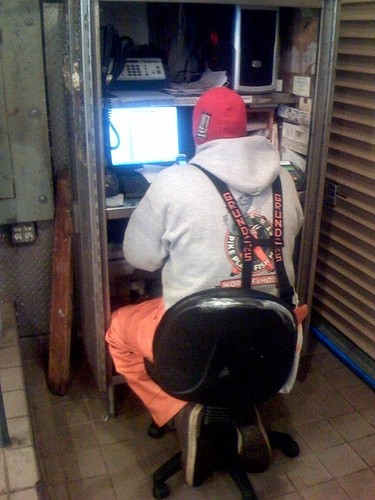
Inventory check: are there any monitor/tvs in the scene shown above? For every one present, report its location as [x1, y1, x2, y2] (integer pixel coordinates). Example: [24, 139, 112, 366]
[103, 107, 185, 175]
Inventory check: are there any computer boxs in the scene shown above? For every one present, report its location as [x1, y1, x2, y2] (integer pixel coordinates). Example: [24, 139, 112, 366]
[183, 2, 281, 94]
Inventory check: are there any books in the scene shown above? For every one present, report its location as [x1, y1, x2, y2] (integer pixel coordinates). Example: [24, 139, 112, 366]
[246, 116, 268, 130]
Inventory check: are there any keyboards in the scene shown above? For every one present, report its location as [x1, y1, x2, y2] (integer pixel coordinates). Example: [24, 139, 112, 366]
[122, 176, 151, 199]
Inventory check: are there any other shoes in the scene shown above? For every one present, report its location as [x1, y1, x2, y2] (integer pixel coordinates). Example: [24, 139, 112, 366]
[174, 403, 206, 488]
[231, 397, 271, 474]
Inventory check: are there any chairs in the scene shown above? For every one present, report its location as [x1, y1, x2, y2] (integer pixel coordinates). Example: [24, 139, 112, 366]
[143, 289, 298, 500]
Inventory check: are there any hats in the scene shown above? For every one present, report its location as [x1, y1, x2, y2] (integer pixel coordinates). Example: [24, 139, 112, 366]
[192, 86, 247, 145]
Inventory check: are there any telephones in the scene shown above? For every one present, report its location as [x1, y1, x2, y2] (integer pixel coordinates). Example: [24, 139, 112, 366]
[106, 36, 170, 91]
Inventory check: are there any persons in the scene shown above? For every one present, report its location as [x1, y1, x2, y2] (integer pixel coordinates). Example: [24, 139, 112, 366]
[104, 86, 309, 474]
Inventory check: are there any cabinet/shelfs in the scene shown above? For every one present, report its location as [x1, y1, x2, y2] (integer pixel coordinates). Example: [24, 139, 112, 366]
[67, 0, 340, 419]
